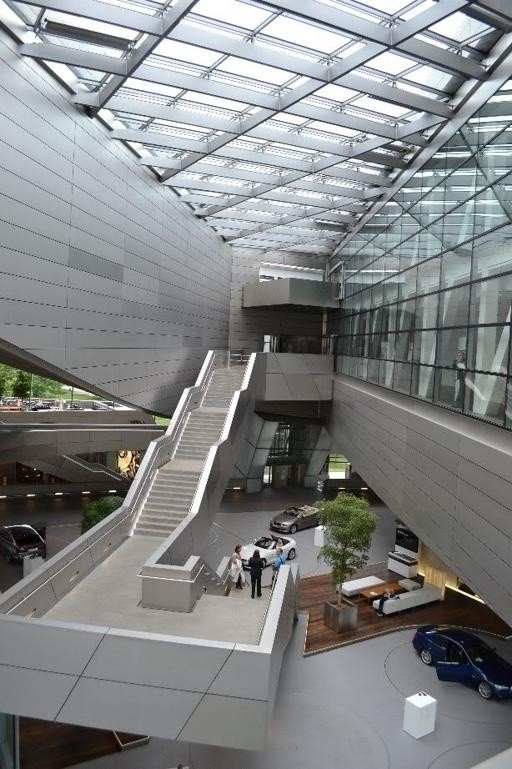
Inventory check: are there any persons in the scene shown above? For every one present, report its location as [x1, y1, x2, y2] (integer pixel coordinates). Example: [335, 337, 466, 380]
[248, 550, 264, 599]
[232, 543, 243, 589]
[271, 548, 285, 587]
[453, 351, 466, 410]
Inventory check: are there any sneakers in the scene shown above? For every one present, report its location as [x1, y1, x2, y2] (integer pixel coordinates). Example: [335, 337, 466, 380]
[241, 588, 243, 589]
[236, 586, 239, 588]
[251, 596, 254, 598]
[258, 594, 262, 597]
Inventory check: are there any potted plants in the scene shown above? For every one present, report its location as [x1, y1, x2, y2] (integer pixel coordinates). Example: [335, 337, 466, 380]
[311, 491, 380, 634]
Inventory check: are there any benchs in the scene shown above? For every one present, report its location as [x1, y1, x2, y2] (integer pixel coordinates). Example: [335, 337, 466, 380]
[398, 578, 421, 592]
[336, 575, 385, 597]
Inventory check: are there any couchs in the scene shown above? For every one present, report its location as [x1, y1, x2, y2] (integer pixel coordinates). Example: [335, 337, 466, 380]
[372, 582, 442, 618]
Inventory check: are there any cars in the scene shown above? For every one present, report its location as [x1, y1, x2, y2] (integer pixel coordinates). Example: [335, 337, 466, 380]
[0, 524, 45, 563]
[412, 624, 512, 699]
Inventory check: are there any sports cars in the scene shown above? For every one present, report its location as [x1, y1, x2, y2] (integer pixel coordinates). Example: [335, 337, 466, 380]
[240, 535, 297, 570]
[270, 504, 323, 534]
